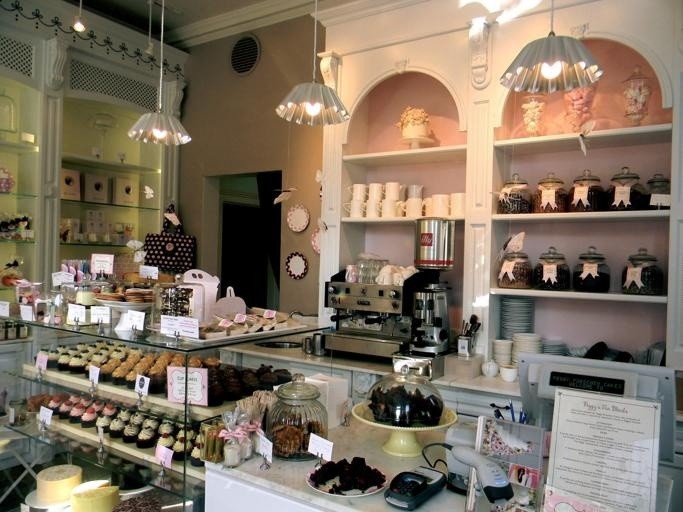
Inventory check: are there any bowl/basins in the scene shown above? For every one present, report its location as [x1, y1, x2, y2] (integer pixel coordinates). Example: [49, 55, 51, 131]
[284, 204, 320, 280]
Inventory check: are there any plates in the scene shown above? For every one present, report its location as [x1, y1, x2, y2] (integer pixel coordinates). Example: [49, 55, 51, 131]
[306, 463, 387, 497]
[25, 478, 155, 512]
[91, 296, 151, 315]
[492, 296, 568, 367]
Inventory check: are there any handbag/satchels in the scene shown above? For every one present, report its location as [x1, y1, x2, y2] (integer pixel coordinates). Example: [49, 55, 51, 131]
[142, 233, 195, 273]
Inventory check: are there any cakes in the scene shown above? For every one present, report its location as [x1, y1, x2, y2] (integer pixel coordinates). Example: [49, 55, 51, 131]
[34, 339, 292, 467]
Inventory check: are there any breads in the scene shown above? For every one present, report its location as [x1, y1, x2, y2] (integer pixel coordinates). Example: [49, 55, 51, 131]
[366, 385, 443, 427]
[112, 496, 161, 512]
[310, 457, 386, 495]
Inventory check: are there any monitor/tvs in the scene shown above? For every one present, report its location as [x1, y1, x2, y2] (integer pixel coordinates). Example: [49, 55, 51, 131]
[510, 352, 678, 464]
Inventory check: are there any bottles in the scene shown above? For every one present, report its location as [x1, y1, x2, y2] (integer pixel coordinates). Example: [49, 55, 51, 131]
[8, 399, 30, 429]
[498, 170, 670, 293]
[266, 374, 329, 461]
[303, 332, 326, 357]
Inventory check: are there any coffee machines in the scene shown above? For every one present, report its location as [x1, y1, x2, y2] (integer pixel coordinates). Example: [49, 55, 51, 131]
[323, 268, 419, 358]
[406, 216, 457, 358]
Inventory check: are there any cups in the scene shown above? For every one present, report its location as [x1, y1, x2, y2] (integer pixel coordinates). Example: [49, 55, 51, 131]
[342, 181, 466, 218]
[457, 335, 474, 357]
[344, 259, 418, 287]
[500, 366, 518, 383]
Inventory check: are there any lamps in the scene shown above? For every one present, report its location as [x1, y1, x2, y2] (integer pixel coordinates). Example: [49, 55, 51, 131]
[274, 0, 350, 126]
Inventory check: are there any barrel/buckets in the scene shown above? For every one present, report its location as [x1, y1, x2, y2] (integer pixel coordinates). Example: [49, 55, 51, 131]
[415, 218, 456, 271]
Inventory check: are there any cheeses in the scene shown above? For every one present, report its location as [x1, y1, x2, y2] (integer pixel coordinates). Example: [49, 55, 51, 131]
[36, 464, 82, 501]
[70, 480, 120, 512]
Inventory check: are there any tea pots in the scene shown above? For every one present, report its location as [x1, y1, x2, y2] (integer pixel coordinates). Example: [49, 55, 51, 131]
[482, 359, 499, 378]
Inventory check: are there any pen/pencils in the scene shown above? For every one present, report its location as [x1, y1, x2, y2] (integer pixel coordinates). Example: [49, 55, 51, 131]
[493, 398, 528, 425]
